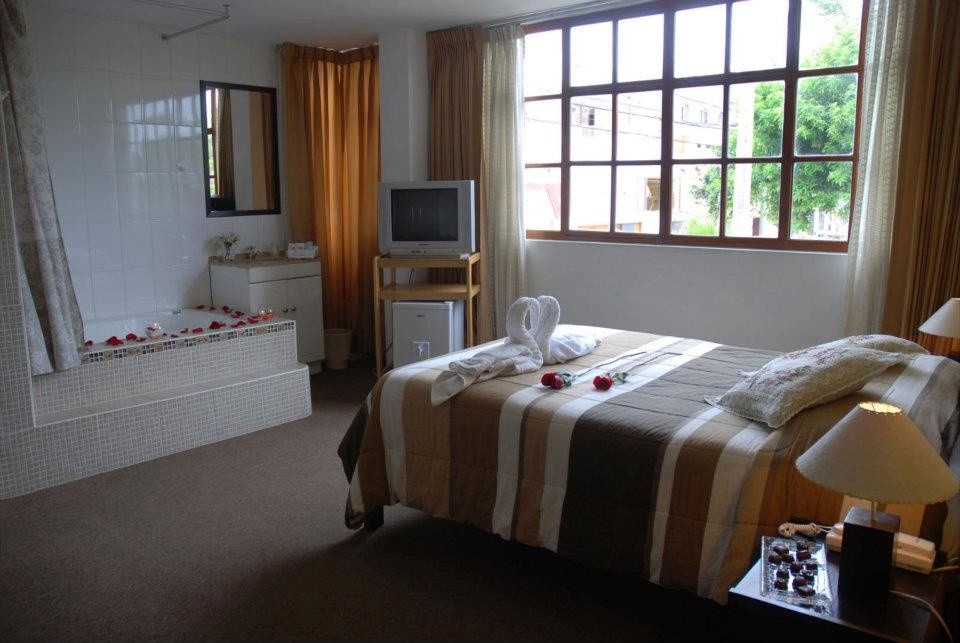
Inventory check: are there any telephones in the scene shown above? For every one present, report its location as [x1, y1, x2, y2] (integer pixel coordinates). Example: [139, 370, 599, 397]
[778, 521, 937, 576]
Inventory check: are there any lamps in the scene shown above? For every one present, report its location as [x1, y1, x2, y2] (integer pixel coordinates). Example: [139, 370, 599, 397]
[792, 403, 959, 599]
[918, 296, 960, 363]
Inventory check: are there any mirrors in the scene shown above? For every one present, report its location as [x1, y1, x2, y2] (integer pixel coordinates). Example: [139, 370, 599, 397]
[199, 80, 281, 217]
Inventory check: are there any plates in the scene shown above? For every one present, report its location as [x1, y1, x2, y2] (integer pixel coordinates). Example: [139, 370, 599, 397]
[760, 533, 834, 608]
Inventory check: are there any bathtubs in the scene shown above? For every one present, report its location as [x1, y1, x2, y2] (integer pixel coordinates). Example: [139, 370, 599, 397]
[31, 305, 297, 417]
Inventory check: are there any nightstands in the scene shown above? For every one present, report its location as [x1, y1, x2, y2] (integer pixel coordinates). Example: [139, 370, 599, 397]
[723, 515, 948, 643]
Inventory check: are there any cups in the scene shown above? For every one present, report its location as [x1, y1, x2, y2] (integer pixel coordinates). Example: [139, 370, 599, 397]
[258, 304, 274, 322]
[144, 320, 164, 339]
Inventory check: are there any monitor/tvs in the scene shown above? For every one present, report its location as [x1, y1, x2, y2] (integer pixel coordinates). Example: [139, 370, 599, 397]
[378, 179, 476, 260]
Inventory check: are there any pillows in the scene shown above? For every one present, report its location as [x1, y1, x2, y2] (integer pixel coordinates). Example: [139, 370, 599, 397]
[703, 346, 905, 431]
[802, 333, 931, 354]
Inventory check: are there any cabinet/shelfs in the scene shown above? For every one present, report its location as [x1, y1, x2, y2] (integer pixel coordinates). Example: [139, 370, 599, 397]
[375, 251, 486, 383]
[210, 255, 326, 377]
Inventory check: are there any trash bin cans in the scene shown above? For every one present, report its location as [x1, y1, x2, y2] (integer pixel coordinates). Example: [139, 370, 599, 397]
[323, 328, 353, 370]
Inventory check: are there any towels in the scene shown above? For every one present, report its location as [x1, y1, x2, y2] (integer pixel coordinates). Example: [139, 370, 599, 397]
[529, 295, 602, 364]
[428, 295, 541, 408]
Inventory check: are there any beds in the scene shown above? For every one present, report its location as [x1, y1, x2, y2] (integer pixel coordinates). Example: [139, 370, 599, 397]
[337, 321, 960, 607]
